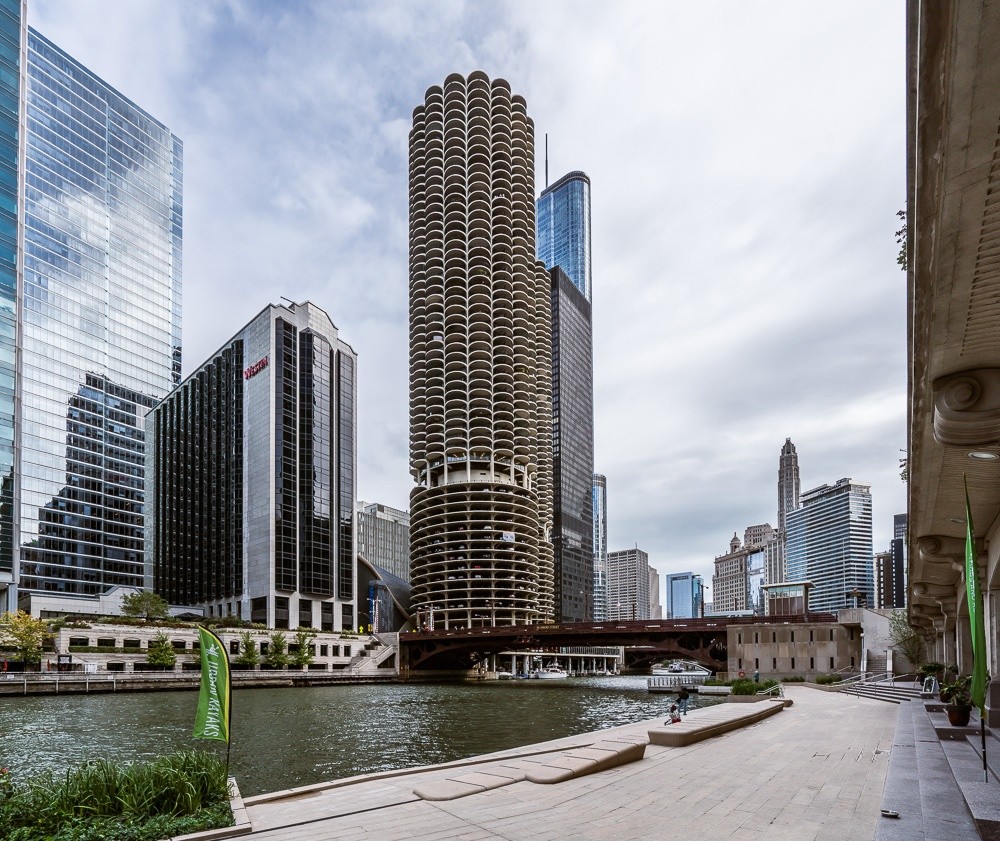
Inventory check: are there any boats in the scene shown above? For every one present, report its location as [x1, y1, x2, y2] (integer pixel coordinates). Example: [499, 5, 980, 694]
[668, 664, 683, 672]
[537, 663, 569, 678]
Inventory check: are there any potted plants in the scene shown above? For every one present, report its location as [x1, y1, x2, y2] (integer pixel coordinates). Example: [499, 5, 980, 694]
[915, 661, 975, 726]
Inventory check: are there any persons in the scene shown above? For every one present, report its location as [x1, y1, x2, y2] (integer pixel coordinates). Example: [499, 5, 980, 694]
[678, 687, 688, 715]
[664, 705, 681, 726]
[753, 670, 759, 683]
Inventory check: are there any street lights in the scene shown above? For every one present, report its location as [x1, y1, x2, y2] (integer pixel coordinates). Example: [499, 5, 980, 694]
[692, 574, 701, 616]
[699, 583, 709, 618]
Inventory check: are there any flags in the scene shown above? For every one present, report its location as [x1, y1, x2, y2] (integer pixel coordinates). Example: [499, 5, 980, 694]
[191, 625, 232, 746]
[959, 471, 989, 712]
